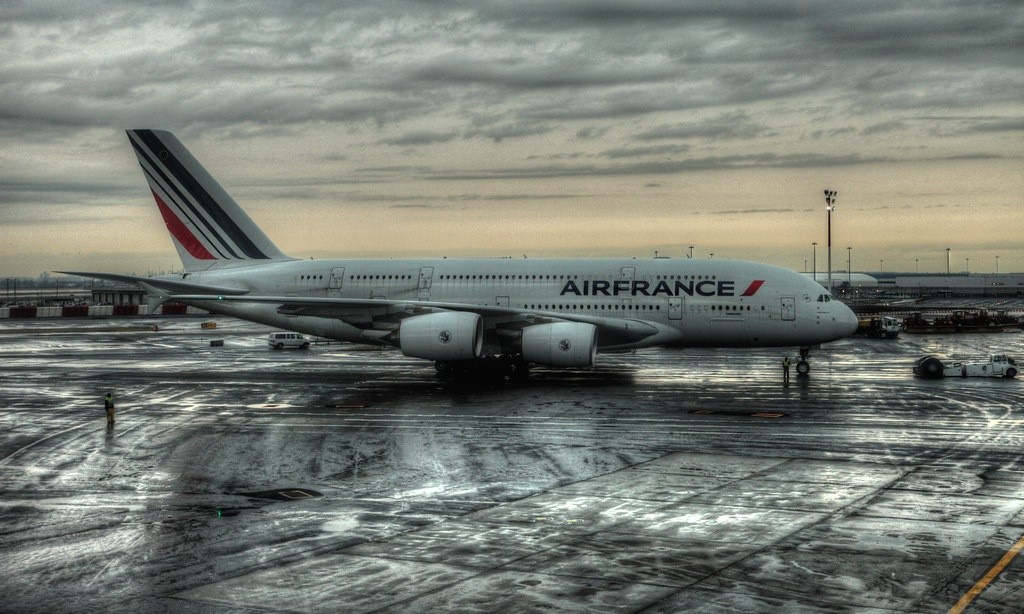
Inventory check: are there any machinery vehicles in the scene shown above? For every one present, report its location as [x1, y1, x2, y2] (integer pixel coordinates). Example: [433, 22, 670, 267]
[911, 352, 1021, 380]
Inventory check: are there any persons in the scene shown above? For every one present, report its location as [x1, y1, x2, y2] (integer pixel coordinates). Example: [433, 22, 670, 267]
[105, 393, 115, 425]
[782, 357, 791, 382]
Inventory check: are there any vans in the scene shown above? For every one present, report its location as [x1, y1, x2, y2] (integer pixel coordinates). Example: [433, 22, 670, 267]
[268, 331, 310, 350]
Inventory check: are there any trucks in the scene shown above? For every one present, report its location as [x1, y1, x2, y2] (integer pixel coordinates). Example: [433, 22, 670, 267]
[855, 315, 902, 341]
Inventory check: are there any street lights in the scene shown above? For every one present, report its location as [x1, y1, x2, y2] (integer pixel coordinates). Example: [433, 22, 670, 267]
[847, 246, 852, 282]
[805, 259, 808, 273]
[946, 247, 951, 273]
[824, 188, 837, 294]
[880, 259, 883, 273]
[915, 258, 919, 273]
[812, 242, 818, 282]
[996, 256, 1000, 274]
[966, 258, 970, 272]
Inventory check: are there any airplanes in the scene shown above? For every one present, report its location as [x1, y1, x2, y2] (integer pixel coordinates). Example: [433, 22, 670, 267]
[50, 128, 863, 390]
